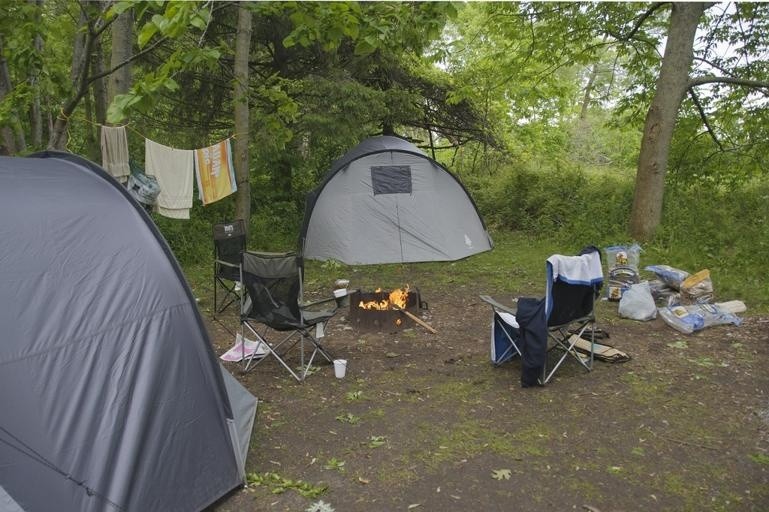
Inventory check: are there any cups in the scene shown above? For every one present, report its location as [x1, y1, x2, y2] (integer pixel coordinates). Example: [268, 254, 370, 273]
[333, 288, 348, 298]
[332, 358, 348, 379]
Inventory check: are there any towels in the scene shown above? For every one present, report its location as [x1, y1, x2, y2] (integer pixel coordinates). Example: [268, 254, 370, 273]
[101, 126, 238, 219]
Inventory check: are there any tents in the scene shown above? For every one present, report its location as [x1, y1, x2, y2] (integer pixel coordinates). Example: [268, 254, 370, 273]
[299, 136, 492, 264]
[1, 147, 261, 510]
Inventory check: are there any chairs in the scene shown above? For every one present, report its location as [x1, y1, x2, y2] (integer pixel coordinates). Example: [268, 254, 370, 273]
[479, 247, 601, 387]
[212, 218, 248, 315]
[239, 249, 337, 383]
